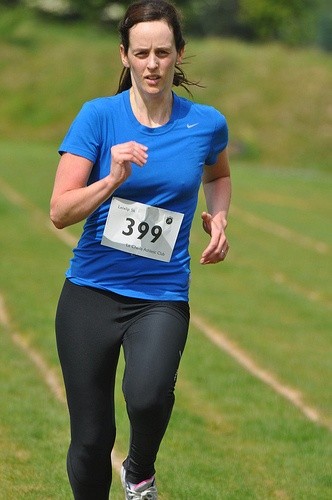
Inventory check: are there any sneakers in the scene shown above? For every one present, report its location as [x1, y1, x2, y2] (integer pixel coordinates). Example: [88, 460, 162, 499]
[119, 466, 158, 500]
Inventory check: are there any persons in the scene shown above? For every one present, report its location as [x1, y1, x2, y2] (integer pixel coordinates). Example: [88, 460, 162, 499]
[49, 0, 233, 500]
